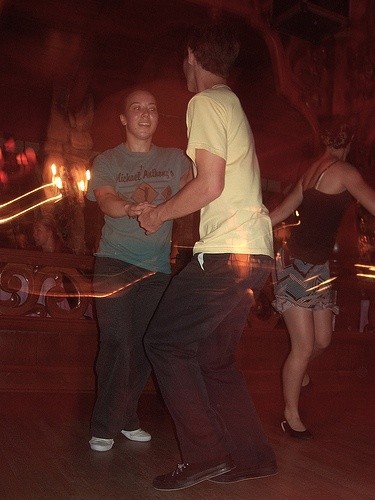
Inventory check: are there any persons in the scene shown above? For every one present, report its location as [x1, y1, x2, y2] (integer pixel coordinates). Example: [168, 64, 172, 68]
[0, 215, 92, 311]
[267, 114, 374, 436]
[81, 83, 196, 452]
[134, 30, 281, 493]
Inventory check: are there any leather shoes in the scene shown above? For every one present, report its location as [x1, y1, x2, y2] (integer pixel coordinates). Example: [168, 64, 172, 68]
[153, 454, 236, 491]
[209, 455, 279, 483]
[302, 378, 313, 393]
[280, 415, 313, 440]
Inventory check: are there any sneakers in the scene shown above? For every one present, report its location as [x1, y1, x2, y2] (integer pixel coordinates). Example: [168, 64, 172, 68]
[121, 428, 151, 441]
[89, 436, 114, 451]
